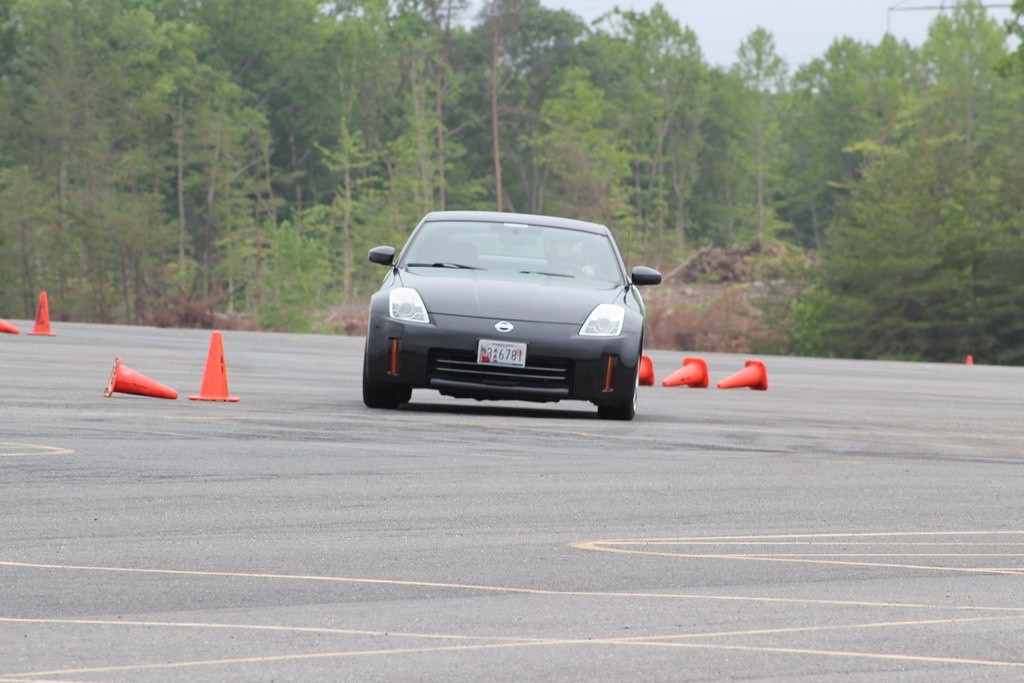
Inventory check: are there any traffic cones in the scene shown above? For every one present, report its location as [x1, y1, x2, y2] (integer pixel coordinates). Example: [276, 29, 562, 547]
[25, 290, 56, 335]
[661, 357, 709, 389]
[965, 354, 975, 366]
[638, 354, 655, 386]
[0, 318, 20, 334]
[187, 330, 240, 403]
[103, 356, 178, 399]
[717, 358, 767, 389]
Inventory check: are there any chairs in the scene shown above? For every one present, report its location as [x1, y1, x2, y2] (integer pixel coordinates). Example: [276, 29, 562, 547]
[441, 241, 482, 269]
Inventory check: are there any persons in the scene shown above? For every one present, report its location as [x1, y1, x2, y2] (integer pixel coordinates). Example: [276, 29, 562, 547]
[527, 232, 596, 280]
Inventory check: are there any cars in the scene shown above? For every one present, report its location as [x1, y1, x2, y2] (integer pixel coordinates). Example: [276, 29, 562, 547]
[363, 209, 663, 422]
